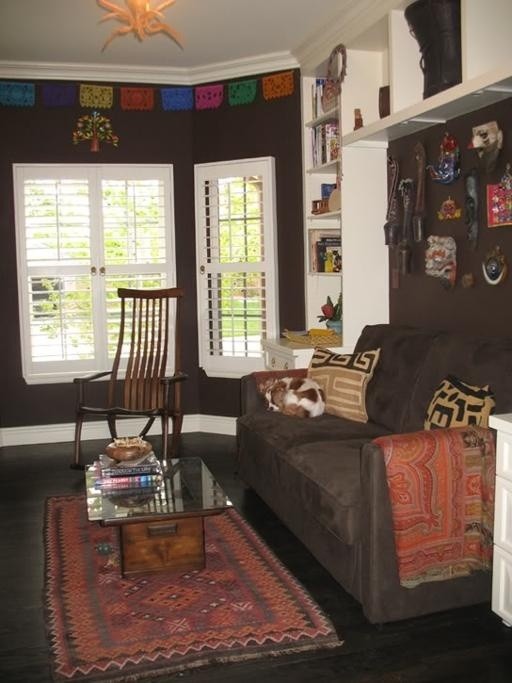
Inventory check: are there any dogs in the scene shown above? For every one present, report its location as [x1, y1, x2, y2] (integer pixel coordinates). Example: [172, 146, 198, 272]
[255, 373, 326, 418]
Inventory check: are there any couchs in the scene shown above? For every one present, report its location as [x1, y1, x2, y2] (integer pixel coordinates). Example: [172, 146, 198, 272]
[235, 324, 511, 624]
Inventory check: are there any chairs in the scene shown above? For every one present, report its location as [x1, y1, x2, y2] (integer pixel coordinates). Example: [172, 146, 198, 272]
[71, 286, 190, 471]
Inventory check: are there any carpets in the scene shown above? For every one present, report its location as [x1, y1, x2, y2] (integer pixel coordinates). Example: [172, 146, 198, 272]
[44, 493, 343, 682]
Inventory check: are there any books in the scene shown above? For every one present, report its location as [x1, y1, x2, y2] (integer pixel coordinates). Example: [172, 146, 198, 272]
[308, 228, 342, 272]
[93, 454, 162, 490]
[310, 79, 339, 165]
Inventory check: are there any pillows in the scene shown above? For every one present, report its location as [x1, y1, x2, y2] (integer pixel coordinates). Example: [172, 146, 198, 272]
[307, 343, 381, 423]
[424, 376, 496, 430]
